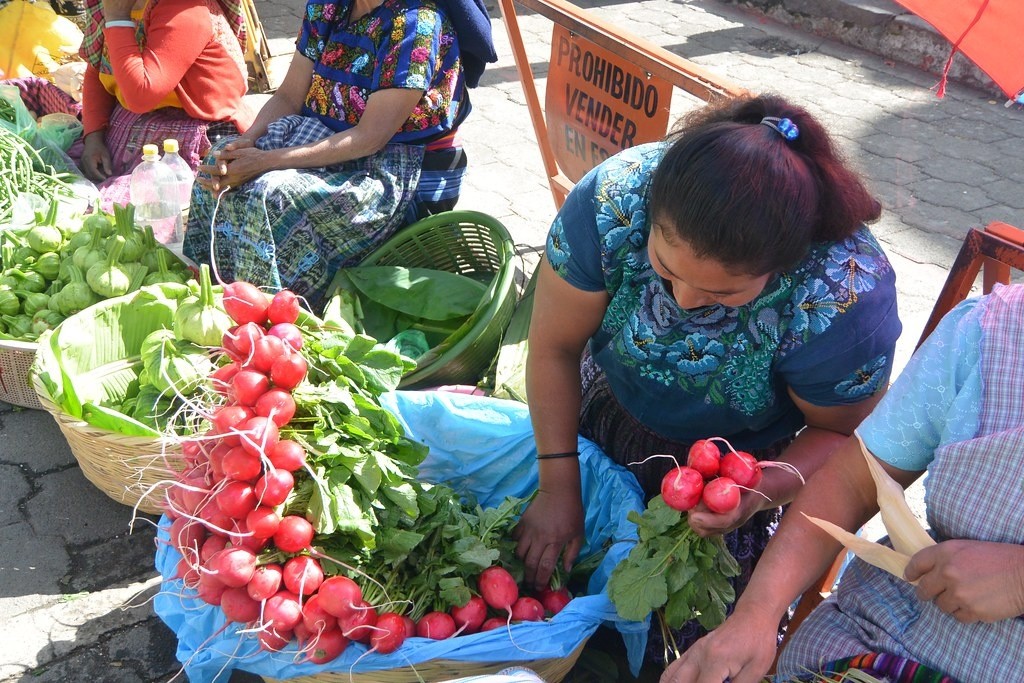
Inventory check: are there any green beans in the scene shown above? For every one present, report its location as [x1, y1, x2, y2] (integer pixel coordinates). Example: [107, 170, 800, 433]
[0, 96, 85, 226]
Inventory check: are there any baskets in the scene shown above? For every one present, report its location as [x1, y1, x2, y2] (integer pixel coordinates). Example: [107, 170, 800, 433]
[323, 210, 517, 391]
[0, 222, 210, 411]
[163, 391, 647, 683]
[31, 283, 320, 516]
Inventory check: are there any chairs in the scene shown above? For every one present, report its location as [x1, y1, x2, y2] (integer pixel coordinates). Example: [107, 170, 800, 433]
[768, 220, 1024, 674]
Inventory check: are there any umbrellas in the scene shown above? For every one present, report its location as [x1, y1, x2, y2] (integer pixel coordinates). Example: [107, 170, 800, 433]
[896, 1, 1024, 101]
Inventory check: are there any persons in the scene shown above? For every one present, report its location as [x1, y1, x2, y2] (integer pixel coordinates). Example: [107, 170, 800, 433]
[660, 281, 1024, 683]
[514, 94, 902, 654]
[182, 1, 472, 317]
[70, 0, 273, 191]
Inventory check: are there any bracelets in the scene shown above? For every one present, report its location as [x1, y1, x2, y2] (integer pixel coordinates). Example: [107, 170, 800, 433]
[535, 452, 582, 458]
[104, 21, 135, 28]
